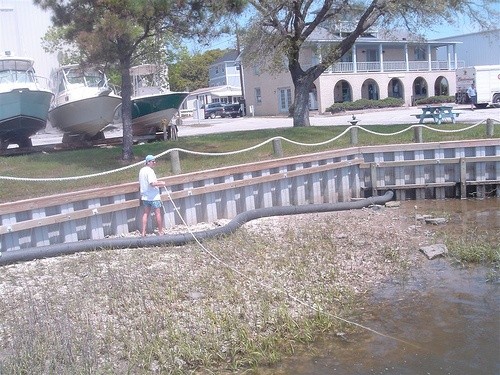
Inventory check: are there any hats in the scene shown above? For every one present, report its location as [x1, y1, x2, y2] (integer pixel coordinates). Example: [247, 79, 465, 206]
[146, 155, 154, 162]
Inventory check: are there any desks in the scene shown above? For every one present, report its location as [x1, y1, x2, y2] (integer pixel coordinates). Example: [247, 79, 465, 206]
[418, 106, 457, 125]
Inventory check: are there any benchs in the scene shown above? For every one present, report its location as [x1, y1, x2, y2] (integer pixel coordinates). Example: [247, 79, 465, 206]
[411, 113, 462, 118]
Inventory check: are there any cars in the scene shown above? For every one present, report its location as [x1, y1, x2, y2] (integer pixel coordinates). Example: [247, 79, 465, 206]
[203, 102, 245, 119]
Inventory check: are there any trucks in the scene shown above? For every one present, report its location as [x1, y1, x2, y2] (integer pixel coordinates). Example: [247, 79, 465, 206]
[473, 65, 500, 108]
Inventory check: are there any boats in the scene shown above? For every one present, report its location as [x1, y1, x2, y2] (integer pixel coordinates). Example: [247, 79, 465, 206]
[51, 64, 126, 143]
[124, 64, 193, 137]
[1, 49, 56, 154]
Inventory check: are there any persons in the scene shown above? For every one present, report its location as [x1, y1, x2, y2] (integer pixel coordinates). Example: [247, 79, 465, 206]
[467, 84, 476, 109]
[139, 155, 166, 236]
